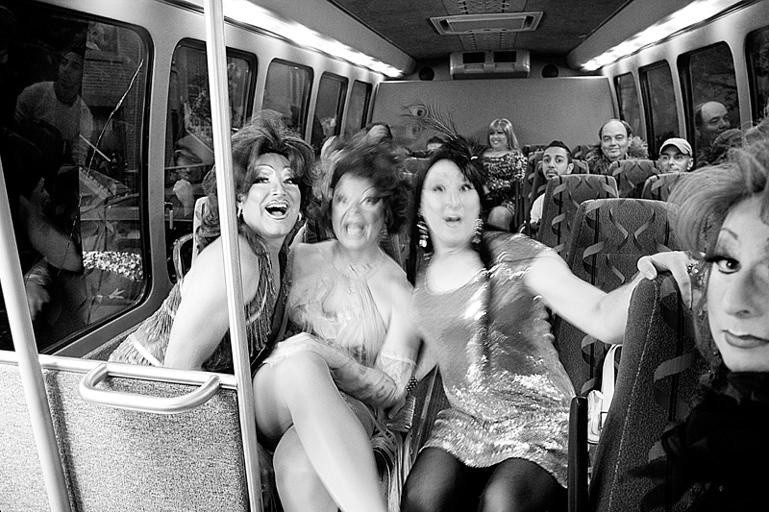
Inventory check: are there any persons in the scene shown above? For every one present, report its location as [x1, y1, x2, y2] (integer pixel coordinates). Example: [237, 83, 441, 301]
[12, 48, 239, 327]
[687, 102, 731, 143]
[104, 108, 769, 512]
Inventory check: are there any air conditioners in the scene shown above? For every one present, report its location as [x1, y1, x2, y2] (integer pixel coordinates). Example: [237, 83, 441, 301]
[447, 50, 531, 79]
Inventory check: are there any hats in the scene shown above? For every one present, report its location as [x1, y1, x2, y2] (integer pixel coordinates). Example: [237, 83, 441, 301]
[659, 137, 693, 157]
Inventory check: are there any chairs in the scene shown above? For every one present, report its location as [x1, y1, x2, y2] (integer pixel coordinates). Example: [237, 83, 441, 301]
[175, 140, 729, 511]
